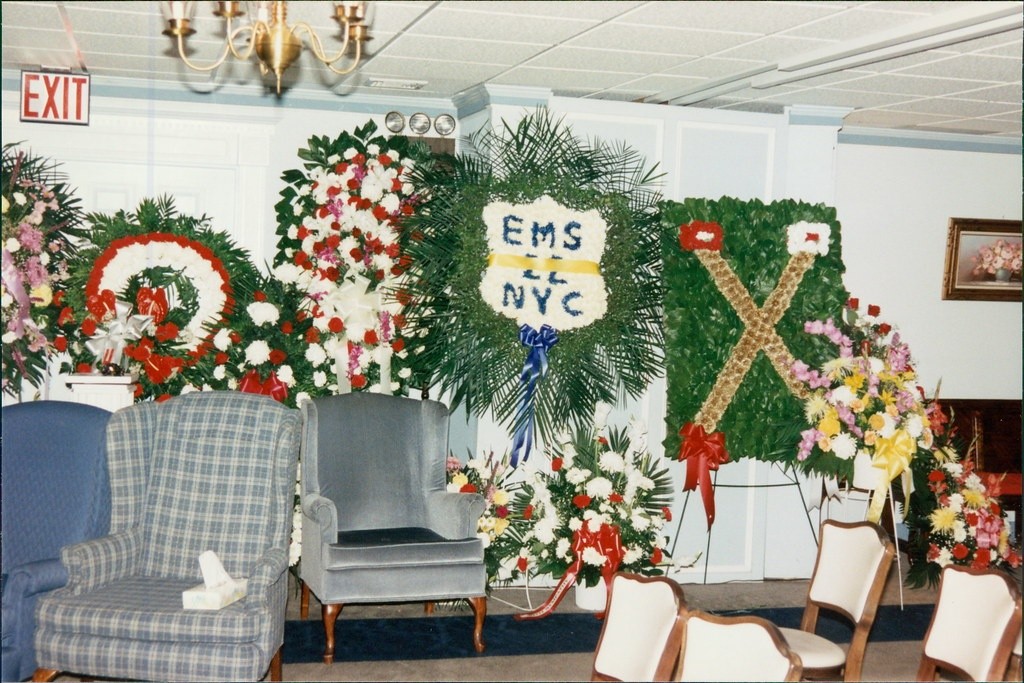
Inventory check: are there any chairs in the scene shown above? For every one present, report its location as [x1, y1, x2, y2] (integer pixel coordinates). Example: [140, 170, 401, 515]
[0, 399, 129, 681]
[590, 571, 685, 682]
[918, 563, 1023, 683]
[879, 397, 1024, 571]
[674, 608, 804, 682]
[302, 392, 485, 661]
[35, 390, 303, 682]
[768, 517, 897, 682]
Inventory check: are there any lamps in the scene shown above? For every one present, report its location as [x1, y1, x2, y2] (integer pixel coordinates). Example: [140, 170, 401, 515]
[164, 0, 376, 96]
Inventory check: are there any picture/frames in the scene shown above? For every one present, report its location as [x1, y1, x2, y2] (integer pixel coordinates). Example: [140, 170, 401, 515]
[942, 217, 1024, 302]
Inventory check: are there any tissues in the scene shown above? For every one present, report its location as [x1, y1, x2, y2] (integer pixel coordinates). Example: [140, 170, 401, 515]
[181, 547, 249, 612]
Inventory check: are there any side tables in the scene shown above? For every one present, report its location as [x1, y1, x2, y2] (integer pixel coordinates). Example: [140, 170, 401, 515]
[65, 370, 140, 412]
